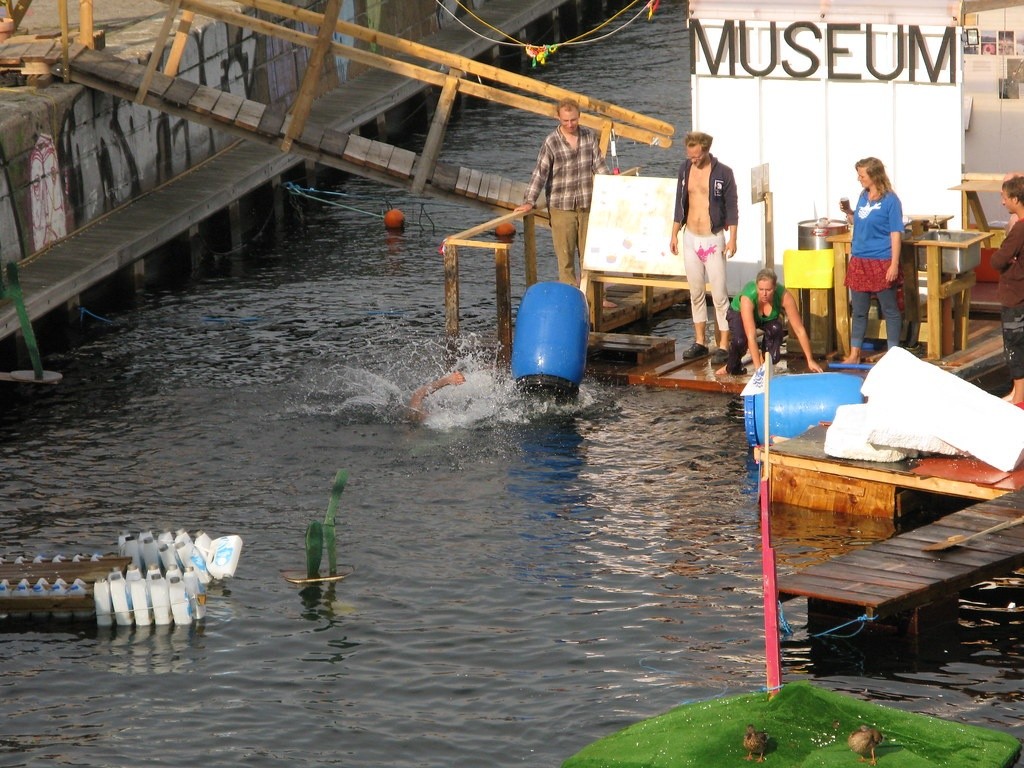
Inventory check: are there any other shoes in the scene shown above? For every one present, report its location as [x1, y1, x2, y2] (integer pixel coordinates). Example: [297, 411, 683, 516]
[1008, 400, 1024, 411]
[727, 359, 742, 374]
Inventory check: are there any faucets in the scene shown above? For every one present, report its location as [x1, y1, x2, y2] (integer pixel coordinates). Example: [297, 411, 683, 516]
[929, 215, 940, 230]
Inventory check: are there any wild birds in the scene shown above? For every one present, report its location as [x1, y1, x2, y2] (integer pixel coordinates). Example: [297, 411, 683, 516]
[743, 724, 769, 762]
[848, 724, 883, 766]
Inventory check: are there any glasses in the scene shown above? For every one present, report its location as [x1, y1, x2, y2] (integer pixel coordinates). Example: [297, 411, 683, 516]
[686, 152, 704, 160]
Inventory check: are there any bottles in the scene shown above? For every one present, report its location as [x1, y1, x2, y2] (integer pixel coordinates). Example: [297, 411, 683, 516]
[0, 528, 243, 626]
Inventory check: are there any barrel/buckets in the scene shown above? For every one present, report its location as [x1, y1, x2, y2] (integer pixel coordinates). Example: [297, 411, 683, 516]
[744, 373, 865, 449]
[511, 280, 590, 397]
[798, 218, 849, 250]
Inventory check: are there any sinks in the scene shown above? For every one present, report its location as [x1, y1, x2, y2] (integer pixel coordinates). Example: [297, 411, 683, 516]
[913, 231, 983, 274]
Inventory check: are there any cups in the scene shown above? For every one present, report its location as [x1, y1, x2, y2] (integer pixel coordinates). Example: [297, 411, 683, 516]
[841, 194, 850, 211]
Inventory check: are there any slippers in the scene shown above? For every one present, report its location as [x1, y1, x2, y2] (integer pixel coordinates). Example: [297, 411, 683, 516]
[683, 343, 709, 358]
[712, 348, 729, 363]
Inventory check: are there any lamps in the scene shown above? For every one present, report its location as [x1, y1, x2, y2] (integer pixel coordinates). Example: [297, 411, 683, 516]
[960, 28, 980, 46]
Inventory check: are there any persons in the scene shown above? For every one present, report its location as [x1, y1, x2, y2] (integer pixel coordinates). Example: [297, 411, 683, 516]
[989, 171, 1024, 405]
[839, 157, 905, 364]
[514, 97, 619, 307]
[669, 132, 739, 364]
[725, 267, 824, 375]
